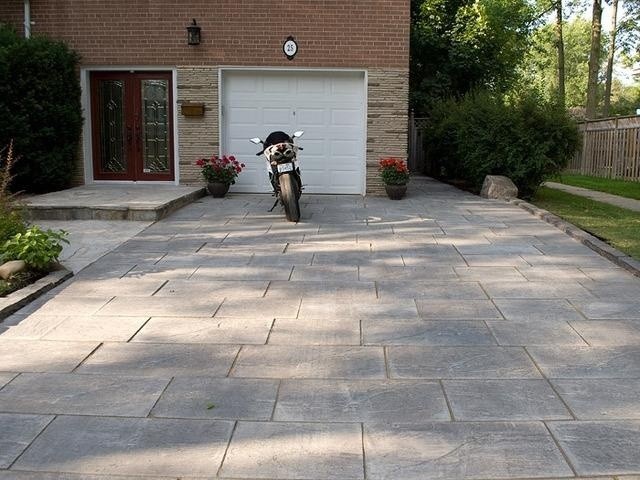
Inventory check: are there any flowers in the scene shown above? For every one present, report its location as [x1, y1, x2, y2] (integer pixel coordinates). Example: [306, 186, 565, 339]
[197, 154, 243, 186]
[379, 158, 410, 185]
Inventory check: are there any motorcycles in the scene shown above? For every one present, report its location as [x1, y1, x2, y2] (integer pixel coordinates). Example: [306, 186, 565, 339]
[250, 129, 304, 221]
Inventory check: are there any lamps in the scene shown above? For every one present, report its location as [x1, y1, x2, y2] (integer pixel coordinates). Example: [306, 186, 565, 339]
[186, 19, 201, 46]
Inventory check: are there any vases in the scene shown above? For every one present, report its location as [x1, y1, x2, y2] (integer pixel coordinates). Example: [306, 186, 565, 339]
[206, 179, 229, 197]
[383, 181, 407, 200]
